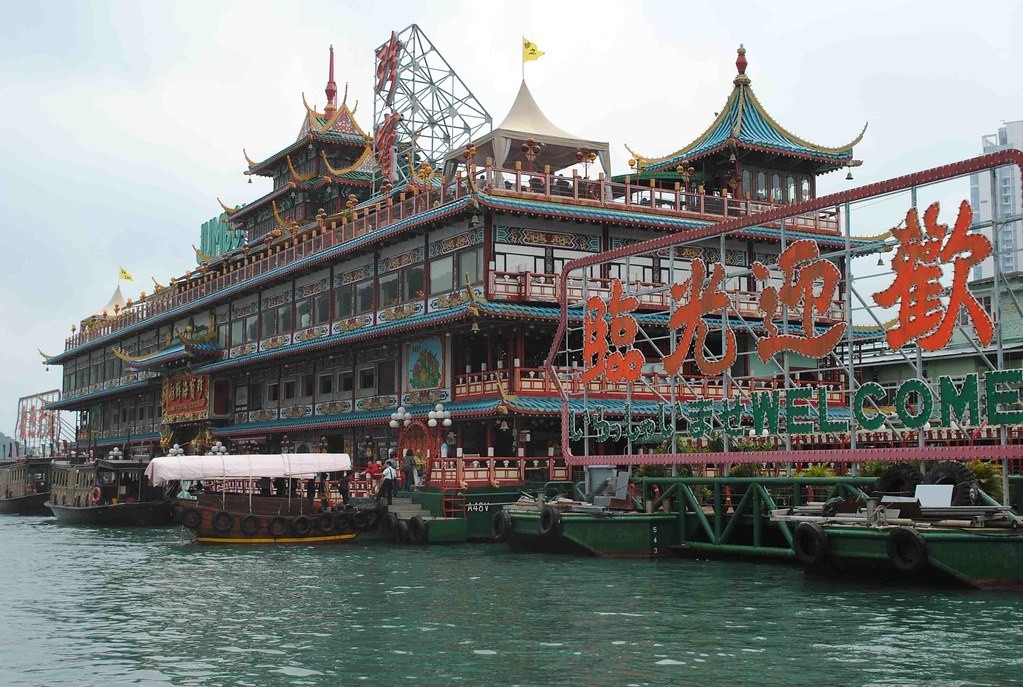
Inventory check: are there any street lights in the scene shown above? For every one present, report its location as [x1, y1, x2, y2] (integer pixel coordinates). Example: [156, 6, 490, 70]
[389, 406, 412, 465]
[427, 403, 452, 458]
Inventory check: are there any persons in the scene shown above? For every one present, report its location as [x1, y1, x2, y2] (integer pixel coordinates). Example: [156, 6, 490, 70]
[258, 448, 424, 509]
[476, 172, 586, 198]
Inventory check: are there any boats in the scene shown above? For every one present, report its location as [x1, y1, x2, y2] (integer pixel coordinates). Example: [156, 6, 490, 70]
[767, 489, 1023, 590]
[0, 442, 54, 516]
[491, 492, 677, 559]
[43, 451, 173, 528]
[142, 452, 388, 543]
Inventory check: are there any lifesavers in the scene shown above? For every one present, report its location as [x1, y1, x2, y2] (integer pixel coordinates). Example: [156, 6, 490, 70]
[91, 487, 101, 503]
